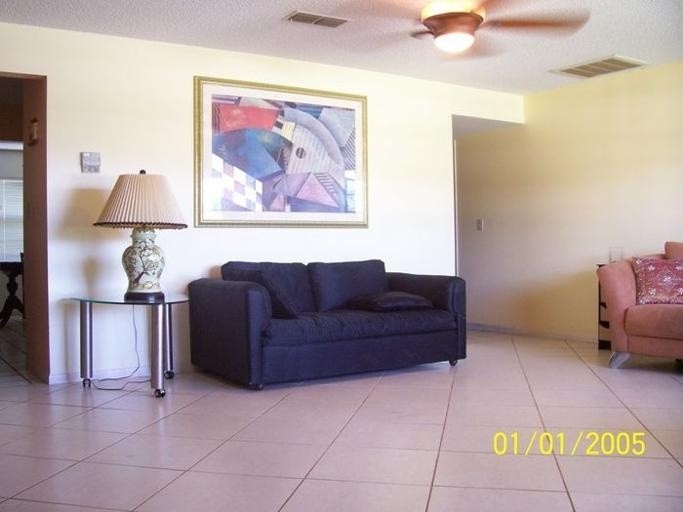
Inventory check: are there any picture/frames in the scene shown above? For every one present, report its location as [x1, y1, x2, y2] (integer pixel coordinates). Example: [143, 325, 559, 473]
[192, 75, 372, 228]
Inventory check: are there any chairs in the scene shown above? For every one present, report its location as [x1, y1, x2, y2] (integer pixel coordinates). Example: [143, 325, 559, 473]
[596, 239, 683, 371]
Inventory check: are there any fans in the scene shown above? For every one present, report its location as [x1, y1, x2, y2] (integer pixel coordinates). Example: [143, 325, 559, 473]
[339, 4, 586, 48]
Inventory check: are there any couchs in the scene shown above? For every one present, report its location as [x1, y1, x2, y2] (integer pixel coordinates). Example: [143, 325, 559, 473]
[189, 258, 468, 391]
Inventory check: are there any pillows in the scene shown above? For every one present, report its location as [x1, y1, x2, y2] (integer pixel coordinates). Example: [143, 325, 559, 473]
[352, 287, 434, 314]
[228, 267, 299, 318]
[629, 252, 683, 304]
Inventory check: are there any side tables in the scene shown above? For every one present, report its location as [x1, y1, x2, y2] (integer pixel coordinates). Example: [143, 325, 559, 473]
[76, 295, 190, 398]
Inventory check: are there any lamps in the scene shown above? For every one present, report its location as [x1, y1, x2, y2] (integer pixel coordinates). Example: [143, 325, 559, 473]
[422, 4, 483, 51]
[92, 172, 187, 302]
